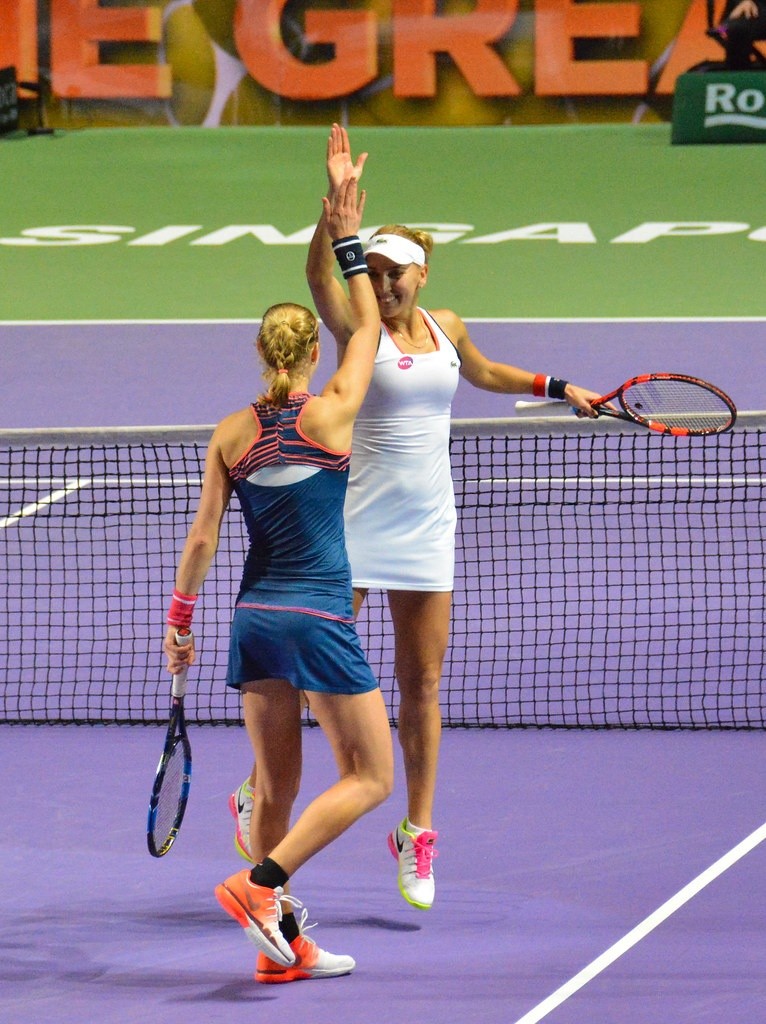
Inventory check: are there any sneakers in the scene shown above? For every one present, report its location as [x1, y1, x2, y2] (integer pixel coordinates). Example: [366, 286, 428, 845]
[214, 869, 304, 967]
[254, 908, 356, 983]
[227, 775, 255, 864]
[388, 818, 439, 911]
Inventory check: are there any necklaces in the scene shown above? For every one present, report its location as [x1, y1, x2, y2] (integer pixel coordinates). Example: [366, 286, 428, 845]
[384, 316, 429, 349]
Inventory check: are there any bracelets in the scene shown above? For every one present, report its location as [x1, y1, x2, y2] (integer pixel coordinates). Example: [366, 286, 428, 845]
[329, 236, 368, 278]
[166, 590, 197, 627]
[531, 373, 569, 401]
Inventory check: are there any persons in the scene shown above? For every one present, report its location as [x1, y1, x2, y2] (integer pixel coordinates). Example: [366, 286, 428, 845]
[161, 171, 396, 982]
[227, 123, 619, 908]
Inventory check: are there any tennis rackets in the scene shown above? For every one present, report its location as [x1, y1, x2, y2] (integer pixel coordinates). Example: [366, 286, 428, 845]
[146, 628, 193, 858]
[514, 372, 737, 437]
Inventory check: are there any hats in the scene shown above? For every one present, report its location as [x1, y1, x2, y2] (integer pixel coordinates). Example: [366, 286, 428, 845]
[363, 234, 426, 268]
